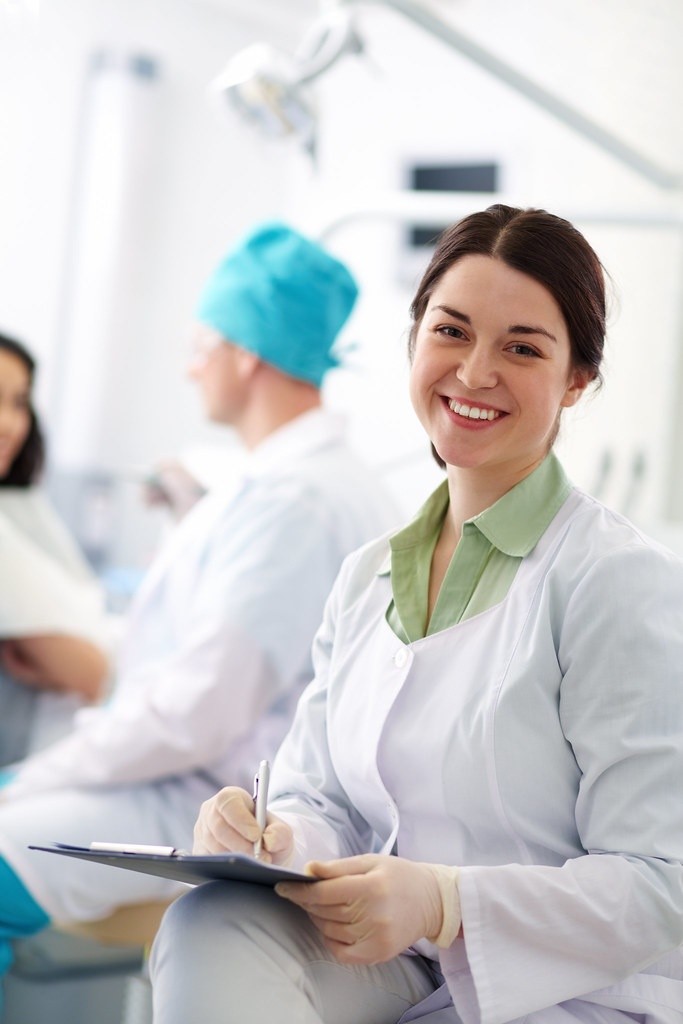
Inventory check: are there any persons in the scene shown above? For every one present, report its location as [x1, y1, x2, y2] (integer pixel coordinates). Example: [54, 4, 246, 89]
[148, 204, 683, 1024]
[0, 336, 109, 701]
[0, 221, 407, 998]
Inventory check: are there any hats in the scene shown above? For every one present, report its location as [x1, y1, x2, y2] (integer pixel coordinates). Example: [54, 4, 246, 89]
[194, 218, 359, 388]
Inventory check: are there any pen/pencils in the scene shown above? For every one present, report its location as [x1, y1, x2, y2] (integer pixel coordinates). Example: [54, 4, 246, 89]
[252, 759, 270, 860]
